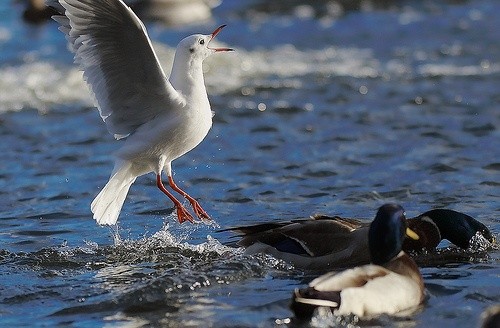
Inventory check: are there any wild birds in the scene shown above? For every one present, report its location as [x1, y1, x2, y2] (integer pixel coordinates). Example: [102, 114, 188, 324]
[213, 215, 374, 271]
[50, 0, 236, 228]
[288, 202, 425, 323]
[401, 207, 496, 255]
[16, 0, 405, 28]
